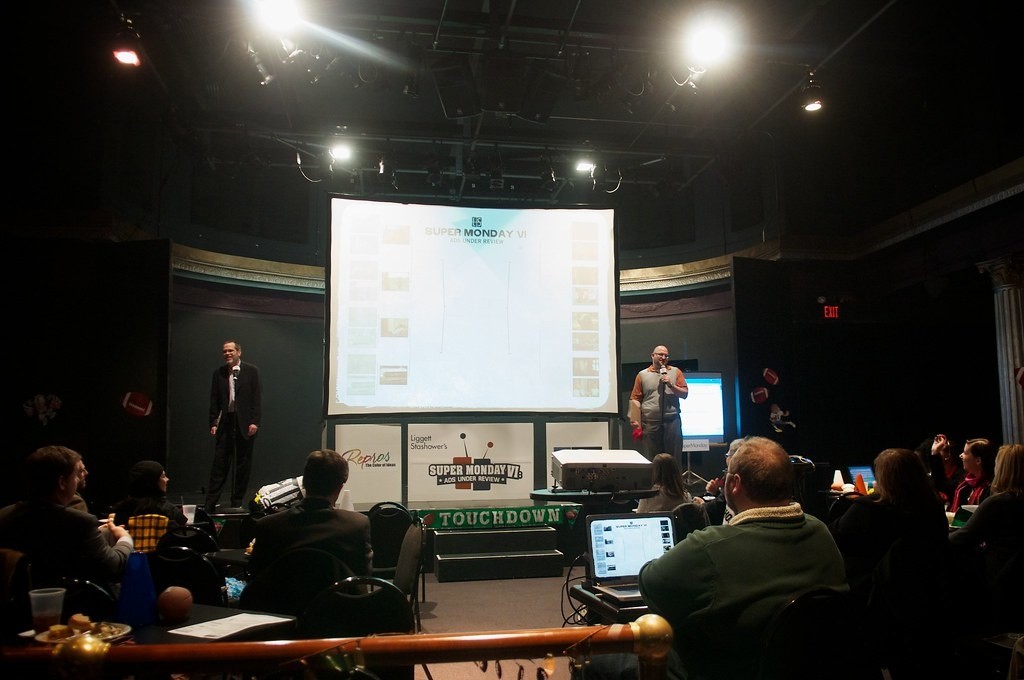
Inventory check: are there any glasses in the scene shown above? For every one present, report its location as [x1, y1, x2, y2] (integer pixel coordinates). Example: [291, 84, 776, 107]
[722, 469, 743, 482]
[653, 353, 671, 360]
[724, 453, 734, 459]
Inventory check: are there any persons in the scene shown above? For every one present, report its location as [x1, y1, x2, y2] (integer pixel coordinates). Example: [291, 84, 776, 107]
[636, 453, 692, 513]
[627, 344, 688, 474]
[199, 338, 261, 515]
[916, 433, 996, 511]
[247, 449, 374, 577]
[0, 444, 134, 624]
[134, 461, 189, 525]
[639, 437, 851, 680]
[948, 444, 1024, 636]
[833, 449, 948, 603]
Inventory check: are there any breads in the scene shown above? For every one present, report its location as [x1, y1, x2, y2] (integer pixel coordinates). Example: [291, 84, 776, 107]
[831, 483, 844, 491]
[48, 613, 93, 639]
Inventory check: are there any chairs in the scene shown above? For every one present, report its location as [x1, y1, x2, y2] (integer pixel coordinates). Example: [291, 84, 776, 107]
[26, 504, 254, 607]
[313, 501, 434, 680]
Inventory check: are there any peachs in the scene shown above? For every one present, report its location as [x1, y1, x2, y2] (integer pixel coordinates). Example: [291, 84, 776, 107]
[159, 586, 193, 619]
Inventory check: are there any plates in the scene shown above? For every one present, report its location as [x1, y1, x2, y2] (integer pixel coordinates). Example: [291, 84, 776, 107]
[18, 629, 38, 637]
[34, 622, 132, 643]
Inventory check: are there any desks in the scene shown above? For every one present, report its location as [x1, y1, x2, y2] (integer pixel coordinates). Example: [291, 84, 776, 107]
[0, 608, 296, 680]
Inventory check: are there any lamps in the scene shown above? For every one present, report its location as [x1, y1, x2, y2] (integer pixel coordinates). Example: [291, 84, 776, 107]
[107, 25, 825, 183]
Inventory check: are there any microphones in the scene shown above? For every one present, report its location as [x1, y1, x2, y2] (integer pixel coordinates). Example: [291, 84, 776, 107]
[233, 365, 240, 382]
[660, 365, 668, 385]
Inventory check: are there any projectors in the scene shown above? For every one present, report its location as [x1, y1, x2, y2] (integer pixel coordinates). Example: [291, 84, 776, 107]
[551, 449, 655, 492]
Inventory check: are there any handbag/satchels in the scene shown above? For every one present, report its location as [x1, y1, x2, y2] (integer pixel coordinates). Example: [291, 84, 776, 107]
[119, 553, 157, 626]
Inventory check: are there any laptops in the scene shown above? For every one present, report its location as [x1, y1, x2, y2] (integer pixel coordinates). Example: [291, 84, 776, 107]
[849, 466, 876, 489]
[585, 511, 677, 603]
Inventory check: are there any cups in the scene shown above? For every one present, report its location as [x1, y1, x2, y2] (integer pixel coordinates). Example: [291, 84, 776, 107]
[183, 505, 197, 525]
[28, 588, 66, 634]
